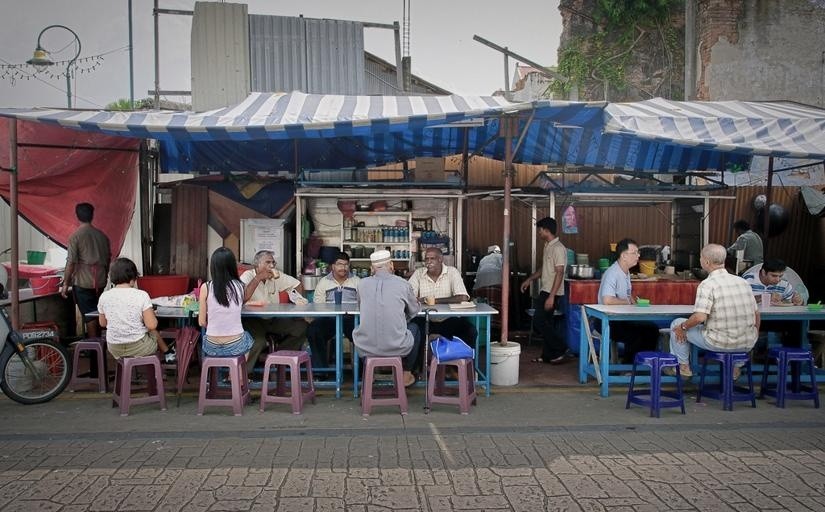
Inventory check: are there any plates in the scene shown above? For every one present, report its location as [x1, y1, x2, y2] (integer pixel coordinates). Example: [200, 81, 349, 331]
[771, 302, 795, 306]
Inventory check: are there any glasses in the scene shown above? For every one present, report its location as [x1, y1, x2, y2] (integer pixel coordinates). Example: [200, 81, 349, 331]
[335, 263, 350, 268]
[626, 250, 640, 255]
[263, 261, 276, 266]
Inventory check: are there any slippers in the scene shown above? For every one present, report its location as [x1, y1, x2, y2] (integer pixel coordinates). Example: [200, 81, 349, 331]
[732, 368, 740, 381]
[405, 373, 424, 389]
[531, 357, 544, 363]
[661, 364, 693, 377]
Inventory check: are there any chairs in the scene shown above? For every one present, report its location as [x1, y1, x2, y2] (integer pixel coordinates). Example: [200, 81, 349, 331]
[135, 274, 190, 352]
[235, 263, 293, 355]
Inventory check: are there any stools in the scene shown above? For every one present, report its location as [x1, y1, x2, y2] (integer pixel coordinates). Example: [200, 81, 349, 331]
[623, 349, 687, 417]
[67, 336, 108, 396]
[196, 356, 249, 419]
[260, 349, 316, 414]
[587, 330, 619, 366]
[698, 347, 752, 408]
[22, 320, 63, 373]
[758, 341, 820, 410]
[111, 355, 168, 418]
[423, 356, 478, 416]
[357, 355, 411, 417]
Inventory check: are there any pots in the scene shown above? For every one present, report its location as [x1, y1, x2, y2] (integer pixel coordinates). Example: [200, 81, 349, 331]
[351, 221, 365, 242]
[692, 267, 709, 280]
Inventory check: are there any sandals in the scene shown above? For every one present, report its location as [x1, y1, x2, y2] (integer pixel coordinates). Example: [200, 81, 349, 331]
[221, 376, 231, 384]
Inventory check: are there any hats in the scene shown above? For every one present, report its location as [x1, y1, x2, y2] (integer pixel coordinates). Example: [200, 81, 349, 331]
[369, 250, 391, 266]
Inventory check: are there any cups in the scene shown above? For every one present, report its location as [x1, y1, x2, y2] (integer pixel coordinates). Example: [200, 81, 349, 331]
[424, 296, 436, 306]
[762, 293, 772, 308]
[270, 266, 280, 279]
[311, 267, 322, 276]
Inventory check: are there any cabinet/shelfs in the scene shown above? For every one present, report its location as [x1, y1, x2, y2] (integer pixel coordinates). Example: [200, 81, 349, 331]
[339, 210, 413, 277]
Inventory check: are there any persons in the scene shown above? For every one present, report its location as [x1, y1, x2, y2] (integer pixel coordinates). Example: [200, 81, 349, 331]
[96, 257, 178, 365]
[352, 248, 421, 387]
[473, 245, 503, 289]
[726, 220, 763, 273]
[304, 252, 367, 380]
[742, 258, 804, 364]
[664, 242, 759, 384]
[521, 216, 569, 362]
[594, 239, 660, 375]
[561, 237, 574, 277]
[199, 246, 256, 389]
[406, 247, 480, 368]
[239, 250, 314, 379]
[60, 202, 111, 377]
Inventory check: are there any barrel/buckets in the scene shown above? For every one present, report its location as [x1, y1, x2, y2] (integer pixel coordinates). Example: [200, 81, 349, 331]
[639, 260, 657, 275]
[490, 341, 522, 387]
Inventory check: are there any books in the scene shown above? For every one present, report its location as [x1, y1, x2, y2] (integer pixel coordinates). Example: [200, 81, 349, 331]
[446, 301, 477, 310]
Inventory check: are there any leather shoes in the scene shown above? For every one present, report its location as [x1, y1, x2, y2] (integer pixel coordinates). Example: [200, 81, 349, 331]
[314, 375, 329, 382]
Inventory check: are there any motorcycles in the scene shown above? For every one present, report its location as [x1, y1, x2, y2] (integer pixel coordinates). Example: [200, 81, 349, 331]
[0, 245, 73, 405]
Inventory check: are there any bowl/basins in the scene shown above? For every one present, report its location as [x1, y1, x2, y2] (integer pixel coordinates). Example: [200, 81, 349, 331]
[637, 299, 651, 307]
[136, 274, 189, 298]
[806, 304, 824, 312]
[569, 263, 595, 279]
[599, 258, 610, 271]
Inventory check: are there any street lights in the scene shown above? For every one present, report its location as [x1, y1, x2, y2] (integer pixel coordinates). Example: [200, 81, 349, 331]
[25, 24, 81, 107]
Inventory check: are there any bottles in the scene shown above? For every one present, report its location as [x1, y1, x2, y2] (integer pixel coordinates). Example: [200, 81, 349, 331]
[392, 249, 410, 259]
[358, 226, 409, 243]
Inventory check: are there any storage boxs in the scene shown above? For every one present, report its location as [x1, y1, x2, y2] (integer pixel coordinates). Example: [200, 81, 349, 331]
[415, 156, 445, 183]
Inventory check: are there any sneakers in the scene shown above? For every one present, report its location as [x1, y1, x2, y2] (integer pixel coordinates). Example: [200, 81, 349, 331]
[165, 346, 177, 364]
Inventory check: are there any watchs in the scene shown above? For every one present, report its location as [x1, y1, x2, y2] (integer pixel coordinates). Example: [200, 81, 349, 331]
[681, 322, 688, 332]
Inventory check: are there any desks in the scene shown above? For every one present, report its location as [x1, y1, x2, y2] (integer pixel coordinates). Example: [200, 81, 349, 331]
[90, 299, 500, 401]
[579, 306, 825, 394]
[1, 285, 76, 355]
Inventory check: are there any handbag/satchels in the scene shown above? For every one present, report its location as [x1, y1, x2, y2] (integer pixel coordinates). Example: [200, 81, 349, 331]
[430, 336, 475, 365]
